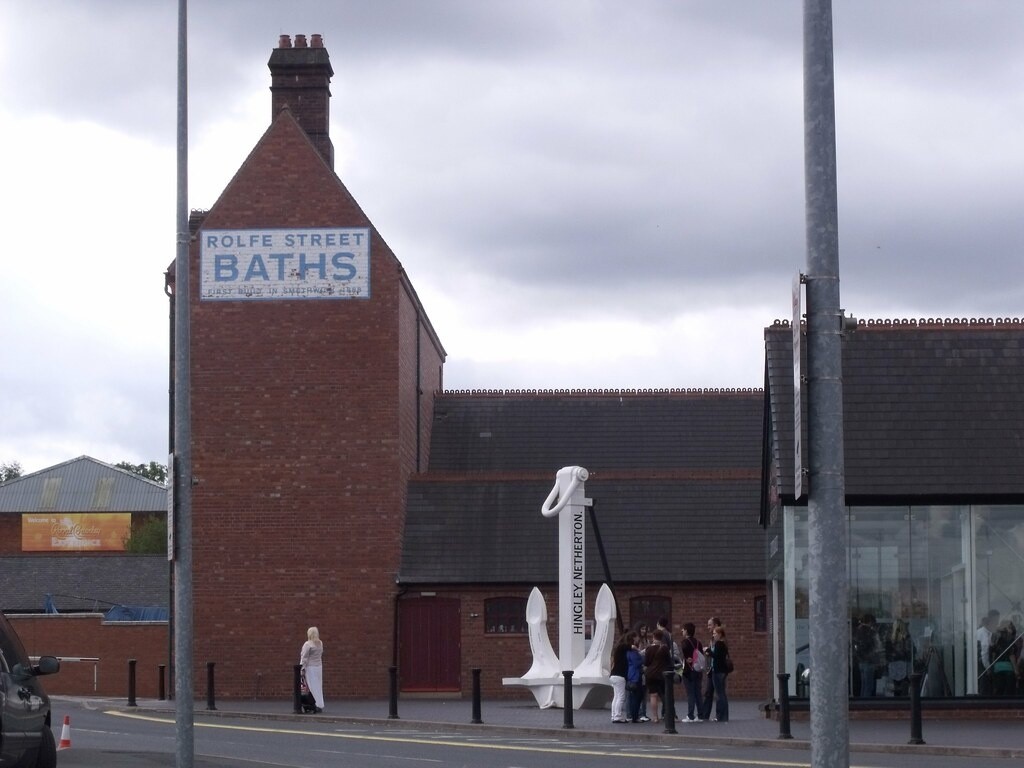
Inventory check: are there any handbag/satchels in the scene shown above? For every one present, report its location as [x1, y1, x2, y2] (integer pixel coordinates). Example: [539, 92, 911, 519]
[707, 667, 712, 678]
[727, 657, 734, 673]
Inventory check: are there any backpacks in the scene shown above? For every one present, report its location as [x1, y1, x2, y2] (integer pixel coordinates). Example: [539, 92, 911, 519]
[691, 649, 706, 672]
[674, 655, 683, 684]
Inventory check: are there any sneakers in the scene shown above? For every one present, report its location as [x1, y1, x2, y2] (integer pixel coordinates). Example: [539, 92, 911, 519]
[682, 716, 693, 722]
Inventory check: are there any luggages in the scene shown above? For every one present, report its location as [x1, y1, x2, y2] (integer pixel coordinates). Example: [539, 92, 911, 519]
[301, 677, 316, 714]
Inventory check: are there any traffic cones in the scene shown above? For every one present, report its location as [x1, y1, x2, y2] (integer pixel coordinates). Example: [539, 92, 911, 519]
[56, 715, 71, 751]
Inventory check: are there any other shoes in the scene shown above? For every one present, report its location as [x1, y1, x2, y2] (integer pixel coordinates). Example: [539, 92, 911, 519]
[714, 716, 728, 722]
[694, 717, 708, 722]
[612, 717, 632, 723]
[654, 719, 659, 723]
[635, 717, 651, 722]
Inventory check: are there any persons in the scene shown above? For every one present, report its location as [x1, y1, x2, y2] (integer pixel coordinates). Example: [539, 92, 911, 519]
[301, 627, 325, 714]
[607, 609, 1024, 724]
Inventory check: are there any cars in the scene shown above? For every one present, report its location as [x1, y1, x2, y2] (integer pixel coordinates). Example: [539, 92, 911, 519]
[1, 612, 58, 768]
